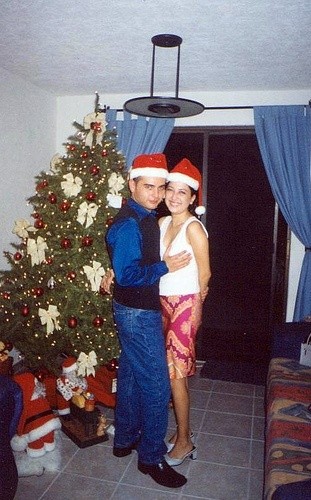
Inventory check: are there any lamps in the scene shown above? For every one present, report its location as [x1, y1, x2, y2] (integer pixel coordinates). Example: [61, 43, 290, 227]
[123, 34, 205, 118]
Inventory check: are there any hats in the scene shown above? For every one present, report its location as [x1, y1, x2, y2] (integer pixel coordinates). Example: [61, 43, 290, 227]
[168, 158, 206, 216]
[129, 154, 171, 180]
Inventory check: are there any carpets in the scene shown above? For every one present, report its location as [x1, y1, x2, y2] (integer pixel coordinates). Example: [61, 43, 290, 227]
[200, 318, 311, 386]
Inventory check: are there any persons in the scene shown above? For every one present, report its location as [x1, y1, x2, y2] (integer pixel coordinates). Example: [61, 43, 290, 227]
[106, 153, 209, 488]
[158, 158, 211, 466]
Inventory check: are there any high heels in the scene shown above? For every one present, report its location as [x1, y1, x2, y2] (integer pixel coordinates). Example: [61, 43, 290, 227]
[164, 446, 198, 466]
[164, 431, 195, 452]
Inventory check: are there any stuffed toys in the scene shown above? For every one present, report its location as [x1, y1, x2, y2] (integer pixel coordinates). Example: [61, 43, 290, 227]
[13, 373, 61, 478]
[58, 357, 88, 417]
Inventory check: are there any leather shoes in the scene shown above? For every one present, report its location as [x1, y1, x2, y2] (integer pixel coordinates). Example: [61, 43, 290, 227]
[114, 437, 140, 458]
[138, 461, 187, 488]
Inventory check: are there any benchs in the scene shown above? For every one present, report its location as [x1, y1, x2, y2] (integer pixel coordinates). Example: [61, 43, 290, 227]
[264, 358, 311, 500]
